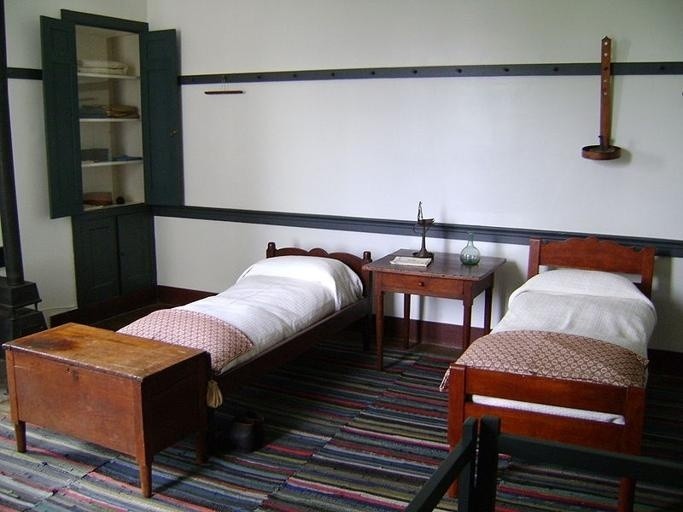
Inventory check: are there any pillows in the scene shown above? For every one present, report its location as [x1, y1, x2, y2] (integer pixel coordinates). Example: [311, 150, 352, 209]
[507, 268, 654, 308]
[237, 255, 364, 311]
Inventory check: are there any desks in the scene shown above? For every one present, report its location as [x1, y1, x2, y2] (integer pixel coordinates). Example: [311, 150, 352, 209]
[1, 323, 213, 499]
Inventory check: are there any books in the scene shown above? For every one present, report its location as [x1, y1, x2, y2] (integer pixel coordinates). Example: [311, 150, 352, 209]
[390, 257, 432, 267]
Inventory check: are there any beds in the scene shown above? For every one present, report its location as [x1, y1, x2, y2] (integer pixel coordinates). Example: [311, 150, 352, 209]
[114, 241, 371, 449]
[448, 233, 656, 512]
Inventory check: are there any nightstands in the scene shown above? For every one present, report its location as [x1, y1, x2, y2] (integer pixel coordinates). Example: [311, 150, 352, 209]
[360, 249, 506, 373]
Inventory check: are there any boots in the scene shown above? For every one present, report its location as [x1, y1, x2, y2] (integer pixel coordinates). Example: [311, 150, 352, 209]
[215, 408, 263, 454]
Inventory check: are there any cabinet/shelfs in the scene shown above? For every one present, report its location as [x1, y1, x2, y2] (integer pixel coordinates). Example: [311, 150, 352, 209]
[37, 7, 185, 323]
